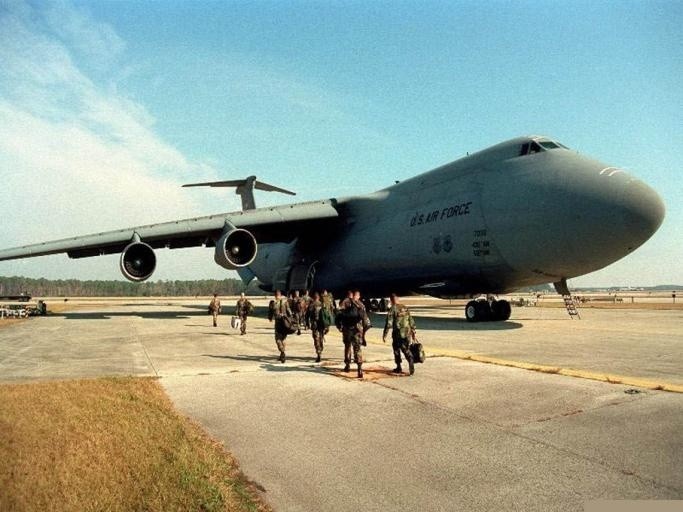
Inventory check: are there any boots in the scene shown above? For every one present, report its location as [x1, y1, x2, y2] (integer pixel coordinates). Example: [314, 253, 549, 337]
[393, 361, 403, 373]
[315, 354, 321, 362]
[408, 357, 416, 377]
[342, 362, 350, 372]
[278, 352, 286, 363]
[296, 318, 313, 336]
[356, 364, 364, 378]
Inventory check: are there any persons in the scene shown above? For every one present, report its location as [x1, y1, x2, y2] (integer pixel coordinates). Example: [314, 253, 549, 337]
[208, 292, 222, 327]
[235, 292, 256, 336]
[267, 286, 418, 378]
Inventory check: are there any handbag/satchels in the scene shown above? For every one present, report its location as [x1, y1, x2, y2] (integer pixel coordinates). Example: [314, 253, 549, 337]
[409, 336, 425, 364]
[281, 314, 298, 335]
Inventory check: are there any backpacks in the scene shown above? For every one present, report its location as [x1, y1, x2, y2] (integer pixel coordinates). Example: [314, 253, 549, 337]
[342, 298, 364, 327]
[317, 303, 332, 329]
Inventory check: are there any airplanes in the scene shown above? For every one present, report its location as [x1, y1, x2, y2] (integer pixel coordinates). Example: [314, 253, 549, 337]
[1, 133, 667, 322]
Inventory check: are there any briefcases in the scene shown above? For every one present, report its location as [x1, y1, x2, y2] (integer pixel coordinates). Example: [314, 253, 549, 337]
[230, 315, 242, 329]
